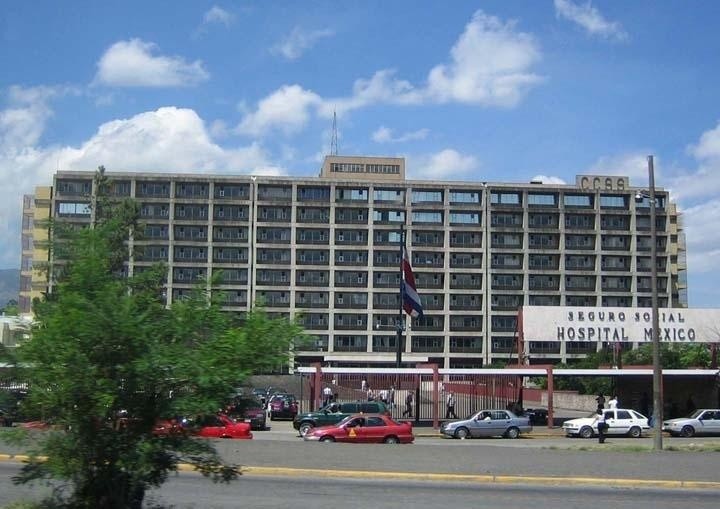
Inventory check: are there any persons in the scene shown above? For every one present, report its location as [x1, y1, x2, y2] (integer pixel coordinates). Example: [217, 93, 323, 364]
[318, 396, 324, 410]
[352, 420, 362, 427]
[589, 409, 604, 443]
[361, 376, 396, 409]
[595, 393, 605, 408]
[608, 396, 618, 408]
[638, 391, 697, 419]
[442, 383, 446, 394]
[513, 401, 522, 416]
[481, 413, 491, 420]
[445, 391, 459, 419]
[324, 385, 332, 403]
[326, 403, 339, 413]
[333, 386, 339, 399]
[402, 391, 414, 417]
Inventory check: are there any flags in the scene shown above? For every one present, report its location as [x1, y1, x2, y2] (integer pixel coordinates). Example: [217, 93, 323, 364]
[401, 233, 423, 321]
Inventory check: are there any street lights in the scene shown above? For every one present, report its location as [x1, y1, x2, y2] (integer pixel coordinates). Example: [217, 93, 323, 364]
[634, 191, 662, 448]
[375, 324, 402, 390]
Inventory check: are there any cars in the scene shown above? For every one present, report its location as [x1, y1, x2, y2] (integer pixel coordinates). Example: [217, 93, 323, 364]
[507, 399, 548, 424]
[439, 408, 534, 440]
[560, 407, 650, 439]
[661, 406, 720, 437]
